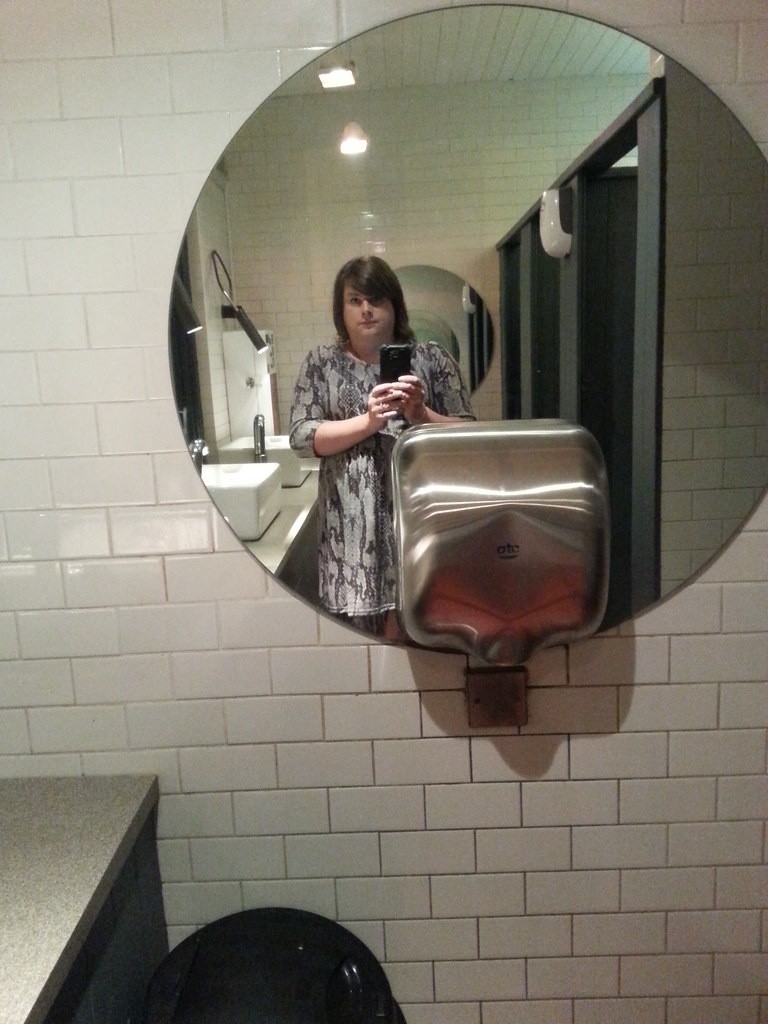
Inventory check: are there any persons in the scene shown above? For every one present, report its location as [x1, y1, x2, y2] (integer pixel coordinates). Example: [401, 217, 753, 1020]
[288, 256, 474, 640]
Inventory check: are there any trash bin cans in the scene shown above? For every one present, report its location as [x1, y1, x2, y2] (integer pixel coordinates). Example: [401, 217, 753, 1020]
[146, 906, 406, 1024]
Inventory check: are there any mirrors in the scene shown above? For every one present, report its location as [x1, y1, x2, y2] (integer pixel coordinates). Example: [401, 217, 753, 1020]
[395, 262, 497, 400]
[163, 2, 768, 657]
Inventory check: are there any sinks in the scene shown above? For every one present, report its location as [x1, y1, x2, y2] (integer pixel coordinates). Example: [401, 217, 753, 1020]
[198, 456, 284, 543]
[219, 432, 312, 487]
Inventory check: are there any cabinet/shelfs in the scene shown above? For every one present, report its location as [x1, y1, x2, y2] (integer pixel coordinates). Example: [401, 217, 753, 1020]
[43, 811, 171, 1024]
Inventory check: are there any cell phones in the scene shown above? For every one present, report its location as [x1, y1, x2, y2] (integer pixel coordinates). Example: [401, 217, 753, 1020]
[379, 343, 412, 400]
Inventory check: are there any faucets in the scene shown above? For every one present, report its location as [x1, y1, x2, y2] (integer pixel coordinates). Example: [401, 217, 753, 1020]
[251, 413, 266, 463]
[188, 436, 209, 474]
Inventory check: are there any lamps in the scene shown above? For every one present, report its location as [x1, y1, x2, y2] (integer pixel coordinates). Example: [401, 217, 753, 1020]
[341, 121, 369, 153]
